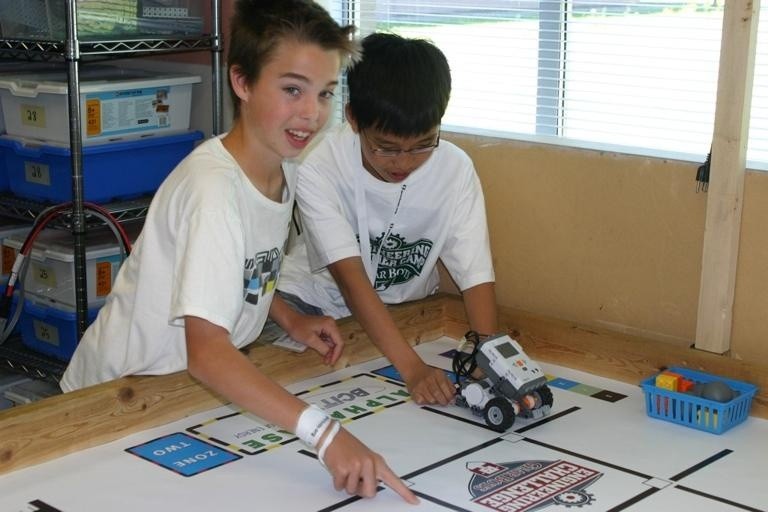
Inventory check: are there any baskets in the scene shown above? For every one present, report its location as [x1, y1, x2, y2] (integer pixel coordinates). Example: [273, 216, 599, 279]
[638, 367, 758, 436]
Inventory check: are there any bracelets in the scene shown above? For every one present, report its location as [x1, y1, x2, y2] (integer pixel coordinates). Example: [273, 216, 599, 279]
[316, 417, 343, 469]
[294, 403, 331, 448]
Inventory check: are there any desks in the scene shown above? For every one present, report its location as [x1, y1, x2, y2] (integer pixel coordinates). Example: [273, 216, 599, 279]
[0, 290, 768, 510]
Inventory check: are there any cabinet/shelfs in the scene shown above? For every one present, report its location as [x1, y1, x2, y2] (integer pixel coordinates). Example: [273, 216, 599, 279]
[2, 1, 221, 384]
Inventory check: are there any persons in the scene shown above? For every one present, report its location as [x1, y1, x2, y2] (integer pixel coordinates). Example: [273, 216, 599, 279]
[246, 31, 501, 406]
[54, 0, 420, 506]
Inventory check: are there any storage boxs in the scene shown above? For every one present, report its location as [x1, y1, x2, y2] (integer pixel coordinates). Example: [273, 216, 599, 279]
[4, 224, 139, 306]
[4, 286, 104, 362]
[3, 2, 205, 41]
[2, 71, 203, 146]
[2, 128, 205, 205]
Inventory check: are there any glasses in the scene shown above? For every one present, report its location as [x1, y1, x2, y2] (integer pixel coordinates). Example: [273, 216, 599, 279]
[362, 121, 440, 158]
